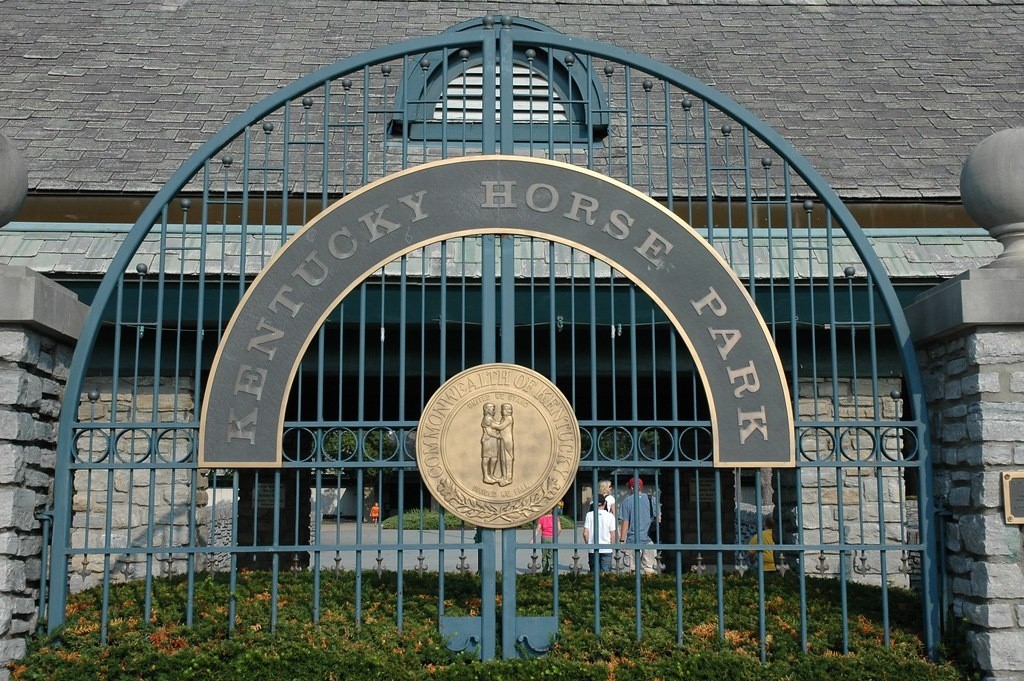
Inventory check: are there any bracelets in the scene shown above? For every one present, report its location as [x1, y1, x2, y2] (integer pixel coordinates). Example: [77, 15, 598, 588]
[619, 539, 624, 542]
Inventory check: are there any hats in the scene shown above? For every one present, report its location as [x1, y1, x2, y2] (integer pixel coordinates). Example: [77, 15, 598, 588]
[598, 494, 605, 505]
[626, 478, 643, 488]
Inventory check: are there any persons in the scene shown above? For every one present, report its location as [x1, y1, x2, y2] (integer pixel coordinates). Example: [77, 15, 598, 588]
[745, 512, 776, 571]
[617, 478, 662, 575]
[529, 507, 562, 574]
[598, 479, 621, 539]
[370, 502, 379, 523]
[583, 493, 616, 572]
